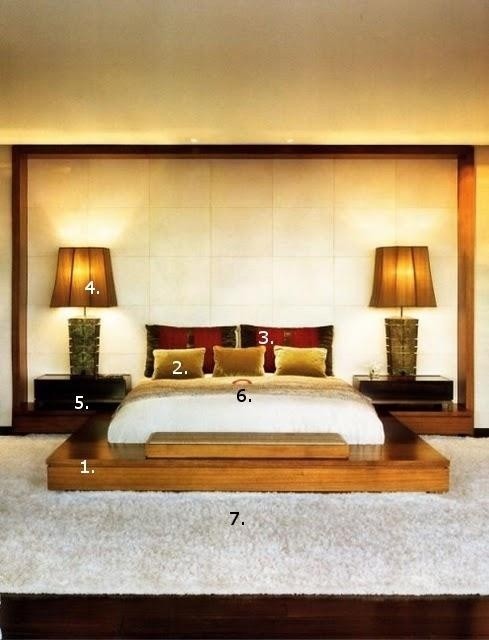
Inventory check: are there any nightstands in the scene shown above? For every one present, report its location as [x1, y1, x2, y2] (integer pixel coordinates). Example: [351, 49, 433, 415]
[353, 373, 454, 407]
[33, 375, 132, 412]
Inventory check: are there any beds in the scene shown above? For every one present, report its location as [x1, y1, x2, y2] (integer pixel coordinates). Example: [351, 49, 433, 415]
[107, 373, 385, 444]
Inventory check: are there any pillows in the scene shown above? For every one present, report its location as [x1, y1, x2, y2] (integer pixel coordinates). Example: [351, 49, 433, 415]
[144, 323, 334, 379]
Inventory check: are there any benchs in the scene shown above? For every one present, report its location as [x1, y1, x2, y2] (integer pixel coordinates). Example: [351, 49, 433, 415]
[144, 432, 350, 462]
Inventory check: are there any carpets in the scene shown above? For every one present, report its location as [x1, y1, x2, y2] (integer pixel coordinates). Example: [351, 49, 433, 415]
[0, 433, 489, 596]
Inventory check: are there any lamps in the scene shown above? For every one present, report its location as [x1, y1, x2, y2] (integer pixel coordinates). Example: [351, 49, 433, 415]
[368, 245, 439, 376]
[50, 246, 118, 375]
